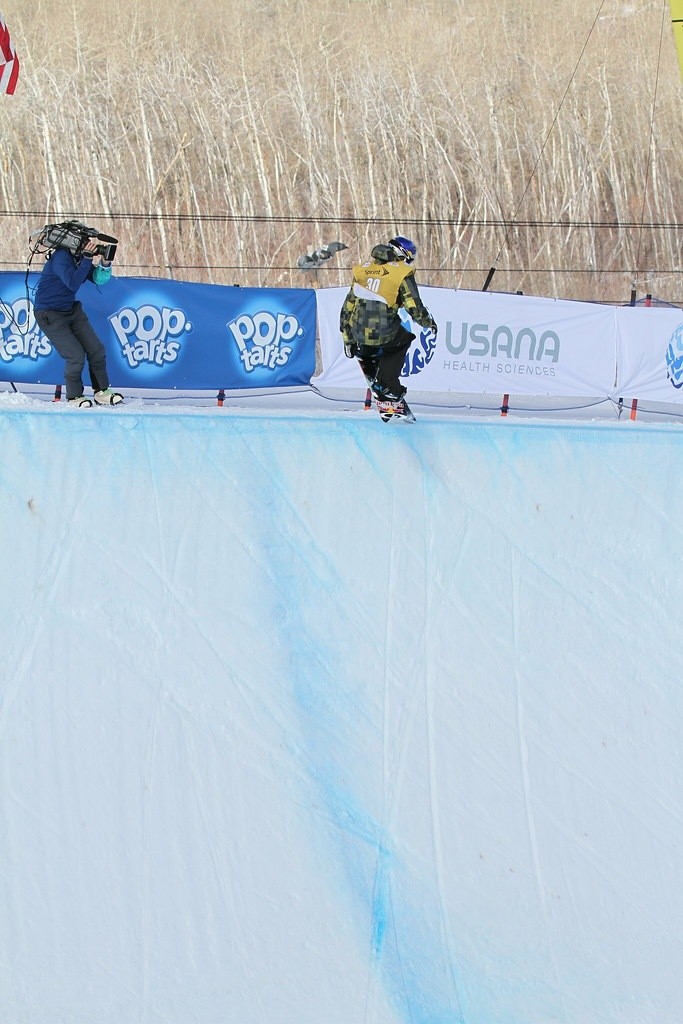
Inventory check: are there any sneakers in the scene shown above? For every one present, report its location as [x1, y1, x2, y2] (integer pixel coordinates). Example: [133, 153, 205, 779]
[372, 383, 400, 400]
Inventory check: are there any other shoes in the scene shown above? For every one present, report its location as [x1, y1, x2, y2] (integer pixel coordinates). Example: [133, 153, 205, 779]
[94, 387, 122, 404]
[67, 396, 90, 408]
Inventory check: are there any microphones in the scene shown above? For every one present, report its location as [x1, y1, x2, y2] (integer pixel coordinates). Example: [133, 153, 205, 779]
[97, 233, 117, 243]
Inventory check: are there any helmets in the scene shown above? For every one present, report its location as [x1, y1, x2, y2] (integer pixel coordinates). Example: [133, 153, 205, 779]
[388, 237, 416, 262]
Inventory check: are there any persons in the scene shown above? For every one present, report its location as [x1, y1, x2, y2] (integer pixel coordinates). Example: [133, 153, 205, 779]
[34, 222, 122, 407]
[340, 237, 438, 423]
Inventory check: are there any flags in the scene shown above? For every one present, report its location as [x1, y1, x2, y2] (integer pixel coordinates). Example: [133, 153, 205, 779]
[0, 12, 19, 95]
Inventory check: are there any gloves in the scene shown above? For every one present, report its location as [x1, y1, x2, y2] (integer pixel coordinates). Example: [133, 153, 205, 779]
[344, 343, 358, 358]
[431, 321, 438, 334]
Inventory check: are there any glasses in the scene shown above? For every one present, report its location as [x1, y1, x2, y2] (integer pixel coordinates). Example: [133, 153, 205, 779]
[406, 258, 413, 264]
